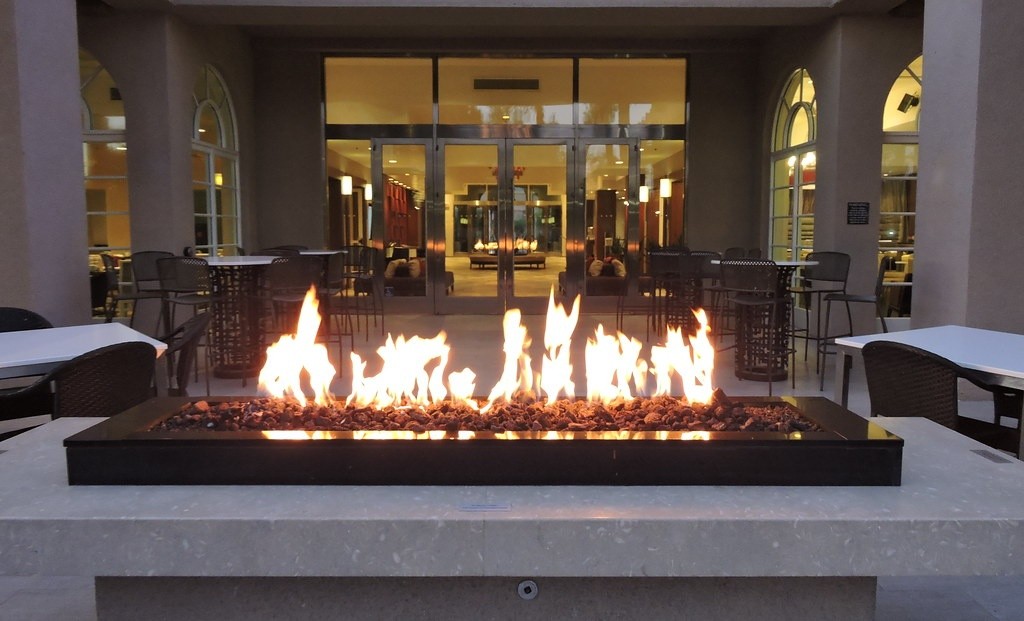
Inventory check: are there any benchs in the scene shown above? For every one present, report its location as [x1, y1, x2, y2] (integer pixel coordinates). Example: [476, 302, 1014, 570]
[469, 253, 545, 268]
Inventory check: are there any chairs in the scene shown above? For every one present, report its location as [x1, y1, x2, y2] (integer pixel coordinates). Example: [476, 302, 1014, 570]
[861, 340, 1023, 458]
[0, 244, 385, 442]
[617, 245, 914, 393]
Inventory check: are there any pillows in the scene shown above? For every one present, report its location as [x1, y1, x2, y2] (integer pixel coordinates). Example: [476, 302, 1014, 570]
[612, 259, 626, 276]
[385, 259, 406, 278]
[408, 259, 421, 277]
[588, 260, 604, 276]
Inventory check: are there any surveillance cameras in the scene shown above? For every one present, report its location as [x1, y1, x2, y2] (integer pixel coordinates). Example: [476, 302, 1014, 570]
[898, 94, 913, 113]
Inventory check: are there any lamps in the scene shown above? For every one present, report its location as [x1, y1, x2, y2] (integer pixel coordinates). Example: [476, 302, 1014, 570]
[660, 176, 672, 197]
[341, 175, 353, 195]
[475, 238, 538, 255]
[639, 186, 649, 202]
[364, 184, 373, 200]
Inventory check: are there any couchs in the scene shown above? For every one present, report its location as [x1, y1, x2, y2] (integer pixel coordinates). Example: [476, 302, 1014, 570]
[353, 257, 453, 296]
[559, 257, 653, 296]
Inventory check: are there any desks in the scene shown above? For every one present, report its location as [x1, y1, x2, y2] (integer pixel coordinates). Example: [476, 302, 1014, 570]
[0, 322, 169, 403]
[199, 256, 289, 378]
[711, 259, 819, 381]
[834, 325, 1024, 462]
[299, 250, 348, 256]
